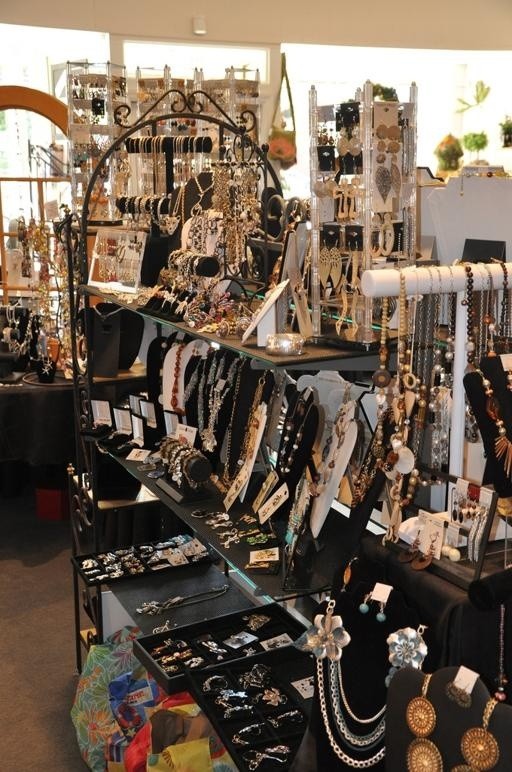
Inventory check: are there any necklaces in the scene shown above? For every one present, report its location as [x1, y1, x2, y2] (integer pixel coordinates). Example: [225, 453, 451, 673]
[0, 60, 512, 772]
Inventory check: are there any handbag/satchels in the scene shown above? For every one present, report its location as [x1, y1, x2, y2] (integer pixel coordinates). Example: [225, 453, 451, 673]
[70, 625, 237, 772]
